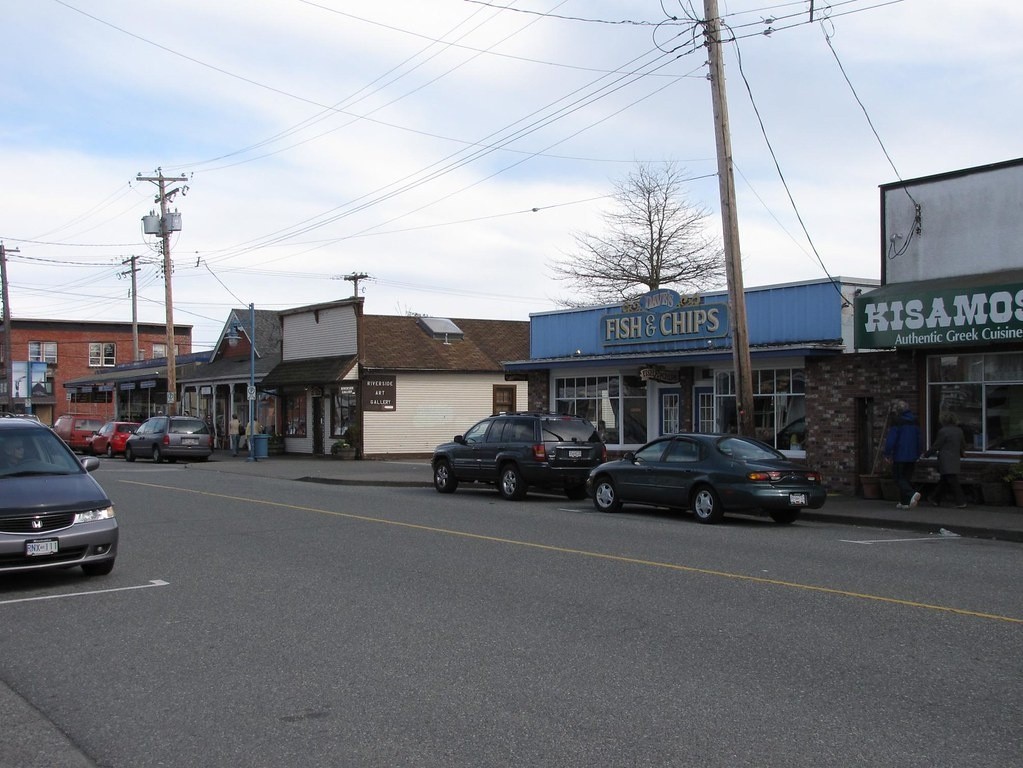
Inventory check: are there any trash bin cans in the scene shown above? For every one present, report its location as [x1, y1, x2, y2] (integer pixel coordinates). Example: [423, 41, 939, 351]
[247, 434, 272, 459]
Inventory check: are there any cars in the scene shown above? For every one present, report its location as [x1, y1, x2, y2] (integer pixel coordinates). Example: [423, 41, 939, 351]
[586, 432, 826, 528]
[0, 416, 119, 576]
[89, 422, 142, 458]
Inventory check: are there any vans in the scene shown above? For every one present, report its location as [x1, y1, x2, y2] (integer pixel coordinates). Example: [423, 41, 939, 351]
[51, 415, 105, 451]
[125, 415, 213, 464]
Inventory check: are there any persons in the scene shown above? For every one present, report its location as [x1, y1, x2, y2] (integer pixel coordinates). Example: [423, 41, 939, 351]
[3, 436, 26, 470]
[881, 400, 923, 511]
[923, 409, 970, 509]
[205, 415, 215, 453]
[245, 417, 263, 457]
[229, 414, 242, 457]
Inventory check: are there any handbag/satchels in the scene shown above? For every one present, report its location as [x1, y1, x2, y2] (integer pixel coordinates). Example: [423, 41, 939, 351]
[239, 421, 245, 435]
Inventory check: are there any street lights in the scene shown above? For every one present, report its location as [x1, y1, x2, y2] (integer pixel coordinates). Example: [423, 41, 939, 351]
[224, 303, 256, 460]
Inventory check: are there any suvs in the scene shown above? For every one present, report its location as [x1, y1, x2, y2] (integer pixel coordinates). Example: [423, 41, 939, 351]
[429, 411, 607, 500]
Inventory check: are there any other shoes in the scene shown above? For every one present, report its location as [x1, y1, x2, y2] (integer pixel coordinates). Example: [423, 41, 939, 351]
[951, 502, 966, 508]
[895, 502, 910, 510]
[908, 492, 921, 510]
